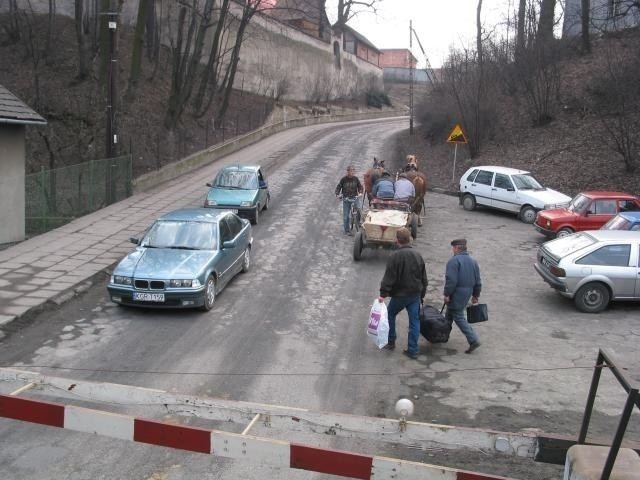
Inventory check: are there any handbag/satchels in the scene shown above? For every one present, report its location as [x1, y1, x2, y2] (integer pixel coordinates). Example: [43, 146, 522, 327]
[467, 304, 488, 323]
[419, 301, 452, 343]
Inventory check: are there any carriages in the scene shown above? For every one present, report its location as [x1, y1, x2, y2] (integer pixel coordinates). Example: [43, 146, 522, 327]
[353, 154, 426, 261]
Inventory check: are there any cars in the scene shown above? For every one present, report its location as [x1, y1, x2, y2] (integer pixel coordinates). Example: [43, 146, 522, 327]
[204, 163, 271, 225]
[457, 165, 573, 224]
[532, 191, 640, 239]
[599, 211, 640, 231]
[533, 229, 640, 313]
[106, 208, 254, 312]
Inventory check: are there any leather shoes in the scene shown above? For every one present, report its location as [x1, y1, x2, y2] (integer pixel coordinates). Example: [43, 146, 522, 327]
[466, 341, 480, 353]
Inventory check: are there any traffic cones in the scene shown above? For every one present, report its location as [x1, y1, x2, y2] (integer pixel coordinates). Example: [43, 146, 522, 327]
[0, 392, 505, 479]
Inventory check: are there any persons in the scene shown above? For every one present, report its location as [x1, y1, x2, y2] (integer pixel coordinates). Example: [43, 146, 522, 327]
[392, 172, 417, 205]
[377, 227, 429, 361]
[335, 165, 364, 238]
[371, 169, 395, 201]
[442, 237, 484, 355]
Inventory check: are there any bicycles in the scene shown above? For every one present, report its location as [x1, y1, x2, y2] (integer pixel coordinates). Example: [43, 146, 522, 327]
[337, 195, 365, 237]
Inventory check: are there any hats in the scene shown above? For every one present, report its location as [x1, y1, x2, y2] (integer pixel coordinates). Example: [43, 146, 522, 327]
[451, 239, 467, 245]
[399, 173, 407, 178]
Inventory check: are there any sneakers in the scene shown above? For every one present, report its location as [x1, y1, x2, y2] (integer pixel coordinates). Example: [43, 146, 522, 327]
[403, 349, 418, 358]
[384, 343, 394, 350]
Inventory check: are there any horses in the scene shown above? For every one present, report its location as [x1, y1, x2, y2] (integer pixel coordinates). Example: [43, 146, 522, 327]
[363, 156, 391, 207]
[402, 153, 427, 227]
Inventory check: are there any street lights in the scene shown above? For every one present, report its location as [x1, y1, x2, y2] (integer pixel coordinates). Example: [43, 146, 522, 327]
[100, 12, 122, 207]
